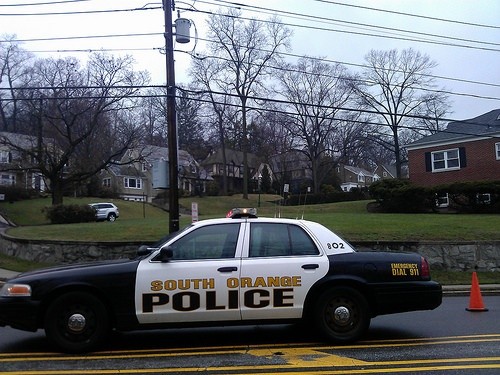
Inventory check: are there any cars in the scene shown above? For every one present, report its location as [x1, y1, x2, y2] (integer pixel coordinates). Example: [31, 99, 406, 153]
[0, 207, 443, 355]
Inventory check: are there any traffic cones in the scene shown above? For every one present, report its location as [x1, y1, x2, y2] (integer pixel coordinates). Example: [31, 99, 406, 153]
[465, 272, 489, 311]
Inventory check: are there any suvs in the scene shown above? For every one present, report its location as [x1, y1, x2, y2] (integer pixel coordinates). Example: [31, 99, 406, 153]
[89, 202, 120, 222]
